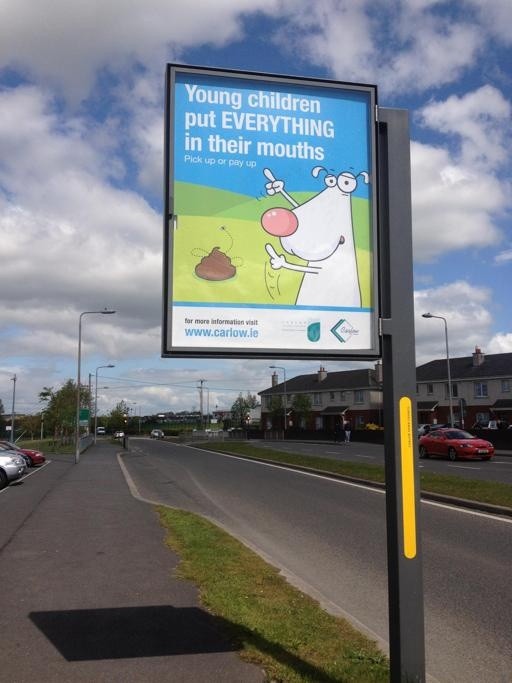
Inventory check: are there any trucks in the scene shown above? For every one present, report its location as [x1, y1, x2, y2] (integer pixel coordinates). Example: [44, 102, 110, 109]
[97, 426, 106, 436]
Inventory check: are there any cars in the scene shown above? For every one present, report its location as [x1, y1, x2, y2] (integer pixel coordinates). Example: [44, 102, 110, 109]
[0, 439, 46, 490]
[150, 429, 165, 439]
[113, 430, 125, 438]
[414, 421, 512, 435]
[418, 428, 495, 461]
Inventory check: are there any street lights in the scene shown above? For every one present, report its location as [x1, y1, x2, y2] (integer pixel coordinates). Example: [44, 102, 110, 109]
[74, 307, 117, 463]
[94, 363, 115, 445]
[421, 312, 454, 428]
[268, 365, 288, 441]
[94, 385, 109, 438]
[9, 373, 17, 445]
[196, 385, 210, 443]
